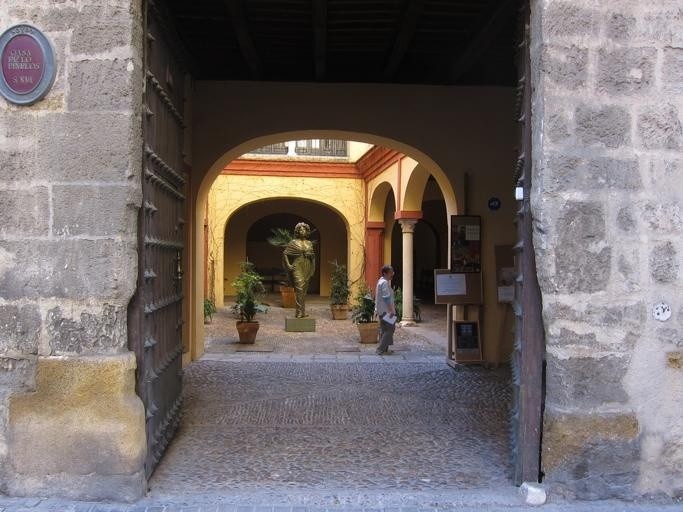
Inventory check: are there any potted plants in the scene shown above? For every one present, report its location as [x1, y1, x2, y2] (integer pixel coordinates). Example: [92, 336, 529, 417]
[351, 277, 380, 343]
[230, 256, 271, 343]
[266, 225, 321, 308]
[326, 258, 355, 319]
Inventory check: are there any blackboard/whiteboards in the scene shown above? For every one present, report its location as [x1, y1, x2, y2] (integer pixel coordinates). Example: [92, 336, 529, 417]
[433, 269, 483, 304]
[454, 322, 482, 364]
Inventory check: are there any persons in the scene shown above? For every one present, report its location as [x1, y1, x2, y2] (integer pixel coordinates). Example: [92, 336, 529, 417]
[283, 221, 315, 319]
[375, 265, 395, 354]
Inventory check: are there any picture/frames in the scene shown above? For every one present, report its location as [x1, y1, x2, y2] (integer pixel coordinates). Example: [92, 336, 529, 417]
[451, 215, 481, 274]
